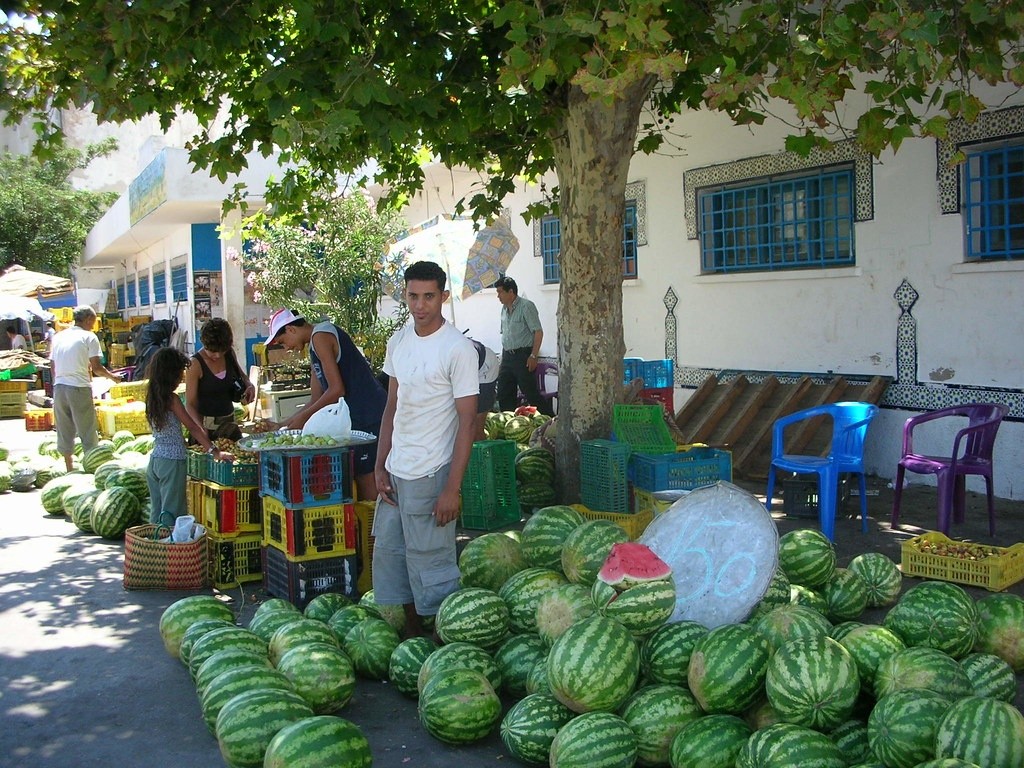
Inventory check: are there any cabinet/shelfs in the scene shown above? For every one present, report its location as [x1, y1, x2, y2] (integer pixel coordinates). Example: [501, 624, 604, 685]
[261, 388, 311, 420]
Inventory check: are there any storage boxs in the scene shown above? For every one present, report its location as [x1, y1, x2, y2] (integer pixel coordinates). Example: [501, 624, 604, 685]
[460, 438, 521, 531]
[184, 446, 376, 608]
[568, 357, 733, 544]
[784, 472, 852, 519]
[901, 532, 1024, 592]
[0, 306, 73, 431]
[89, 311, 187, 437]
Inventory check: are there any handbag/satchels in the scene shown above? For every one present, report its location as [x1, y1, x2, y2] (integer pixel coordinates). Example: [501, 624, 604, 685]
[230, 348, 247, 402]
[124, 511, 209, 590]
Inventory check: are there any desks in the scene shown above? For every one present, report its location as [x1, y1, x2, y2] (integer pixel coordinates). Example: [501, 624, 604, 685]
[252, 343, 309, 382]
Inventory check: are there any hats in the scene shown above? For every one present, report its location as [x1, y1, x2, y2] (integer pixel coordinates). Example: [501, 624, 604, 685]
[46, 322, 51, 325]
[262, 308, 307, 346]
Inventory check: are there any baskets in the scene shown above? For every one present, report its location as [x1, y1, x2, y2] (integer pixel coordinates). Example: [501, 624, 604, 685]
[0, 380, 27, 417]
[901, 531, 1024, 593]
[571, 404, 732, 542]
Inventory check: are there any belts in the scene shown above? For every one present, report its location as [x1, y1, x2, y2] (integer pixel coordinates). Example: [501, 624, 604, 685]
[507, 348, 530, 354]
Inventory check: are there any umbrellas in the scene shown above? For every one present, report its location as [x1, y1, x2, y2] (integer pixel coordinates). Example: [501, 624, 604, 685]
[375, 212, 520, 328]
[0, 264, 72, 352]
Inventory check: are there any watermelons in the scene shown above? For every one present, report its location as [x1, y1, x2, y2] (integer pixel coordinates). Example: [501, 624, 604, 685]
[484, 405, 555, 514]
[233, 402, 250, 421]
[160, 507, 903, 768]
[0, 425, 190, 539]
[867, 580, 1024, 768]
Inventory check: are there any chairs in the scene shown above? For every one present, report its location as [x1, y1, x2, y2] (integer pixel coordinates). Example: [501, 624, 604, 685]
[890, 403, 1010, 536]
[519, 362, 559, 414]
[766, 402, 880, 543]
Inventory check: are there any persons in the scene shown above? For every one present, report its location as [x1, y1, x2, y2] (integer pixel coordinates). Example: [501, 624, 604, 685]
[263, 309, 388, 501]
[145, 348, 235, 526]
[49, 305, 122, 473]
[466, 337, 499, 442]
[6, 326, 27, 351]
[371, 261, 480, 644]
[46, 322, 55, 336]
[186, 317, 255, 447]
[494, 277, 556, 418]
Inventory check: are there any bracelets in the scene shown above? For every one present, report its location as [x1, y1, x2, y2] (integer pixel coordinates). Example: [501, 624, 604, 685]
[208, 445, 216, 453]
[530, 354, 536, 358]
